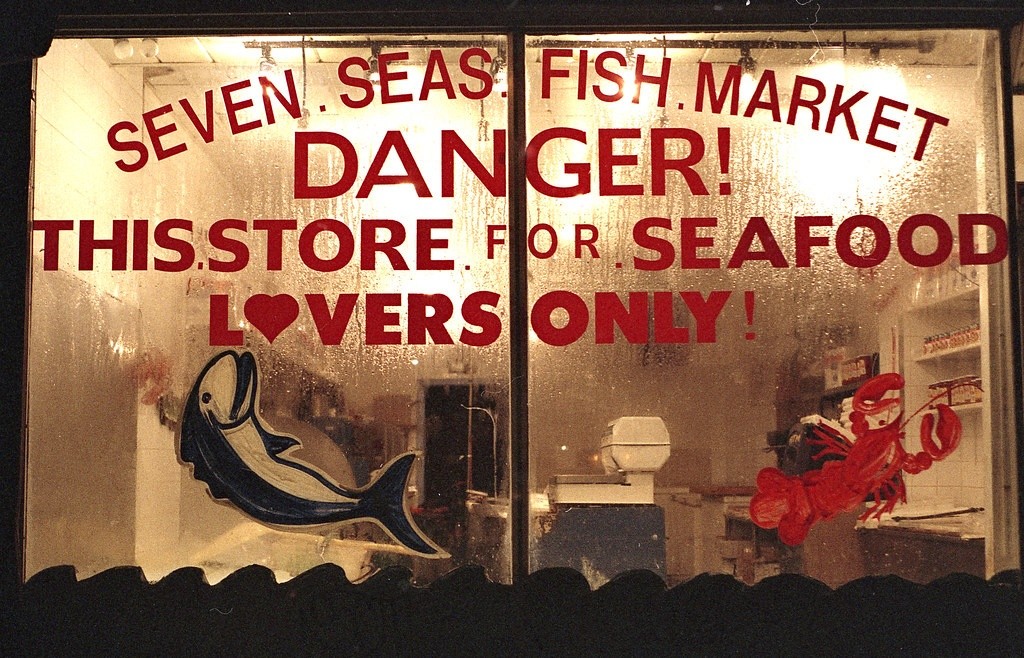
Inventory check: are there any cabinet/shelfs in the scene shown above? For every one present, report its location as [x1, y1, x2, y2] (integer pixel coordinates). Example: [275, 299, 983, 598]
[904, 284, 985, 416]
